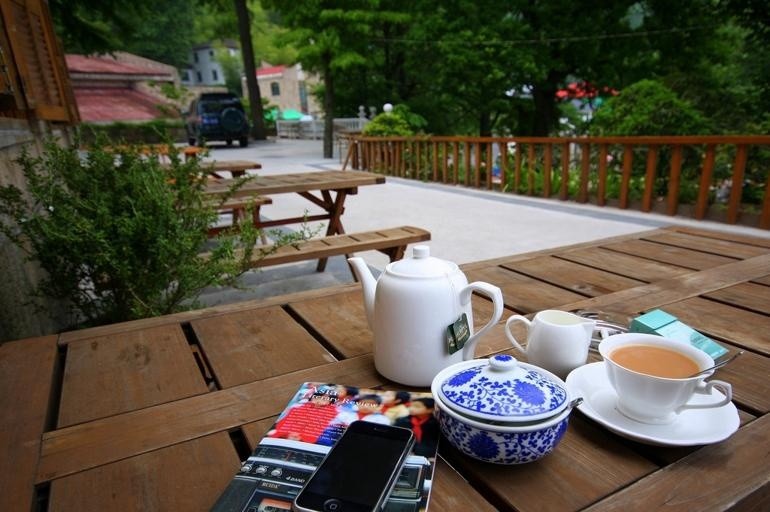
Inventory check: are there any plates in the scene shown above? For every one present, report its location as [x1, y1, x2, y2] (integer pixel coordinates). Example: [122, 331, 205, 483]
[566, 361, 742, 447]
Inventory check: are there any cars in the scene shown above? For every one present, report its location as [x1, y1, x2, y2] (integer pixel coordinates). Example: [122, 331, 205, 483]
[185, 92, 249, 146]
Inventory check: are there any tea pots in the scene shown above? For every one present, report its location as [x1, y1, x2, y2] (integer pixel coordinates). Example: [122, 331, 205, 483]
[349, 246, 504, 389]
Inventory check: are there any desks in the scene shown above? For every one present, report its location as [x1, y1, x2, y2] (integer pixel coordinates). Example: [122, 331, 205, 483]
[0, 223, 768, 510]
[149, 158, 264, 180]
[157, 167, 387, 282]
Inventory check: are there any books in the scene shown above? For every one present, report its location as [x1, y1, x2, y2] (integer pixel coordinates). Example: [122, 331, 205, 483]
[210, 381, 440, 512]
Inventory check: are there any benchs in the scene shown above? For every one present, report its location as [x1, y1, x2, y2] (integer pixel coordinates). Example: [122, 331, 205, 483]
[177, 224, 429, 267]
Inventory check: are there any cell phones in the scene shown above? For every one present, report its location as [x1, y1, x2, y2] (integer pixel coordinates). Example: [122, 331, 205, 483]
[292, 420, 416, 512]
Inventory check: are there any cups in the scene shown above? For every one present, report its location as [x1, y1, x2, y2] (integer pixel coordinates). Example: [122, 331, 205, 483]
[432, 356, 572, 468]
[599, 332, 733, 426]
[506, 309, 597, 382]
